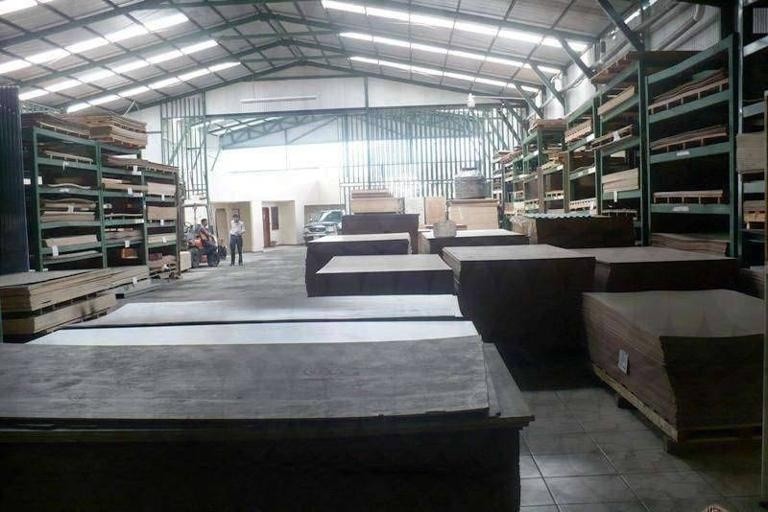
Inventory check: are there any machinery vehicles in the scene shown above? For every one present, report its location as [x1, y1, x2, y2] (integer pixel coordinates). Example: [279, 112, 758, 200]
[183, 222, 220, 268]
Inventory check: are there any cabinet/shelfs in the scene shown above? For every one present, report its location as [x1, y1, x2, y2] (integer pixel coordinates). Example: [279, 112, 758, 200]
[488, 0, 768, 267]
[20, 126, 183, 279]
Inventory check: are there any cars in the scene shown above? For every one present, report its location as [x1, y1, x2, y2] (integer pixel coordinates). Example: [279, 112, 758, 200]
[304, 210, 345, 246]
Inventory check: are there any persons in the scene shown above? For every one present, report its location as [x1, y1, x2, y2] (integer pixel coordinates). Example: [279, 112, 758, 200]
[230, 213, 246, 266]
[198, 219, 218, 251]
